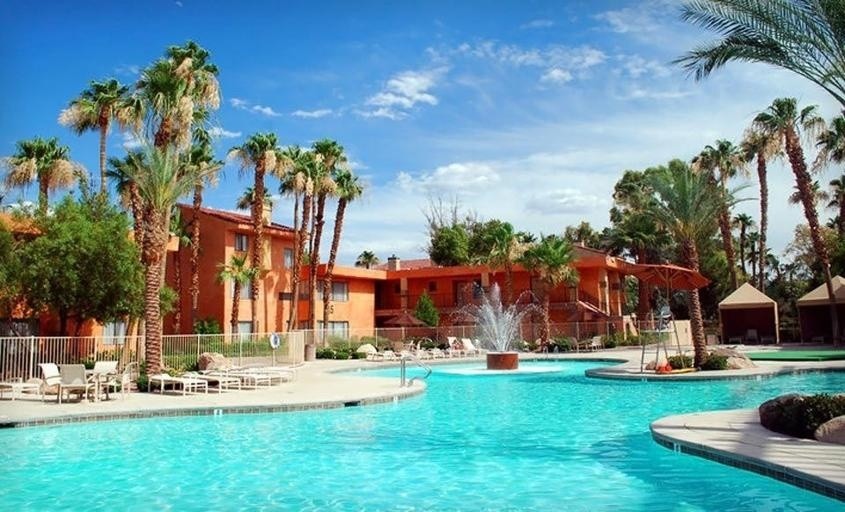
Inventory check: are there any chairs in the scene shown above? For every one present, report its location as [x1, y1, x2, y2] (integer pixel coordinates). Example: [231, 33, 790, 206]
[1, 359, 123, 405]
[147, 362, 303, 395]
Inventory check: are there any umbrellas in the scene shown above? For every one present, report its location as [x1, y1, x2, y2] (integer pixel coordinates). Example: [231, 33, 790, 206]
[384, 310, 425, 339]
[630, 259, 712, 304]
